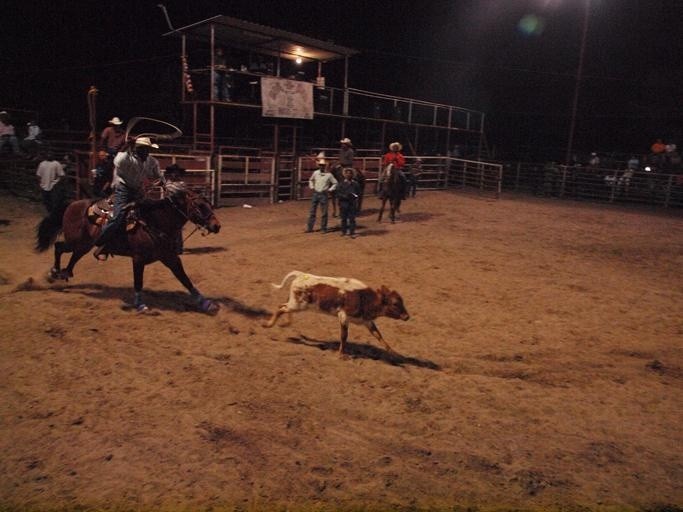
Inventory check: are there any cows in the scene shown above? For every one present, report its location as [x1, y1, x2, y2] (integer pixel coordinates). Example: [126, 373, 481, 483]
[260, 270, 410, 361]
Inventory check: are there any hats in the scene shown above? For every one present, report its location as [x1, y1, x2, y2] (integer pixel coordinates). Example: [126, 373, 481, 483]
[340, 138, 352, 147]
[135, 137, 159, 149]
[342, 167, 358, 179]
[108, 117, 123, 125]
[390, 142, 402, 151]
[316, 158, 330, 165]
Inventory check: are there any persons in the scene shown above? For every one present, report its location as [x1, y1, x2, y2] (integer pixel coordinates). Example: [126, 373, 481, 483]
[337, 138, 353, 168]
[36, 149, 65, 212]
[336, 168, 361, 239]
[382, 142, 405, 172]
[93, 138, 160, 260]
[20, 118, 43, 158]
[305, 159, 340, 233]
[98, 118, 129, 152]
[0, 111, 22, 158]
[213, 47, 232, 103]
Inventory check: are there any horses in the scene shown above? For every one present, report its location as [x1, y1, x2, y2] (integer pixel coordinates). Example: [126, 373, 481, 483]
[31, 188, 221, 314]
[375, 159, 404, 223]
[326, 165, 366, 217]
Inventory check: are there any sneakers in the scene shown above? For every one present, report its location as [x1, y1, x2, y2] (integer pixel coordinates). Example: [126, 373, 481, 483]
[96, 246, 107, 260]
